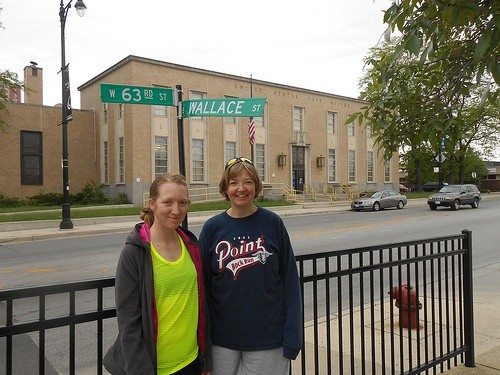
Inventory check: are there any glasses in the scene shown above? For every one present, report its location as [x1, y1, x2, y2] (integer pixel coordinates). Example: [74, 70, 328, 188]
[225, 157, 254, 170]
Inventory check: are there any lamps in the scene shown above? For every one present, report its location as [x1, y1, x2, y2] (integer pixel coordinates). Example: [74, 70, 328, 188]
[317, 155, 325, 170]
[278, 153, 287, 170]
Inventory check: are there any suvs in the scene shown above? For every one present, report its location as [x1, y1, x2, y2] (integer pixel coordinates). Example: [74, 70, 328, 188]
[428, 184, 482, 211]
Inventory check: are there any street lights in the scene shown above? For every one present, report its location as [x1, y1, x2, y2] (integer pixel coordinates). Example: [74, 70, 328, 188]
[59, 0, 89, 229]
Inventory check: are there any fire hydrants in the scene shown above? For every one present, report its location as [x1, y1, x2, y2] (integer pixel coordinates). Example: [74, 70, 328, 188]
[388, 284, 423, 329]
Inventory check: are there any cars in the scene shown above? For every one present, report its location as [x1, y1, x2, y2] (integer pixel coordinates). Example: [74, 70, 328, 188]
[351, 190, 407, 211]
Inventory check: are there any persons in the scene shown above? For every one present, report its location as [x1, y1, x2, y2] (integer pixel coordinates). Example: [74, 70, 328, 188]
[103, 173, 206, 375]
[198, 157, 303, 375]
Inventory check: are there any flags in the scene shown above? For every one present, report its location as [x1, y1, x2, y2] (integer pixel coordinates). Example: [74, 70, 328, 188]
[248, 116, 255, 146]
